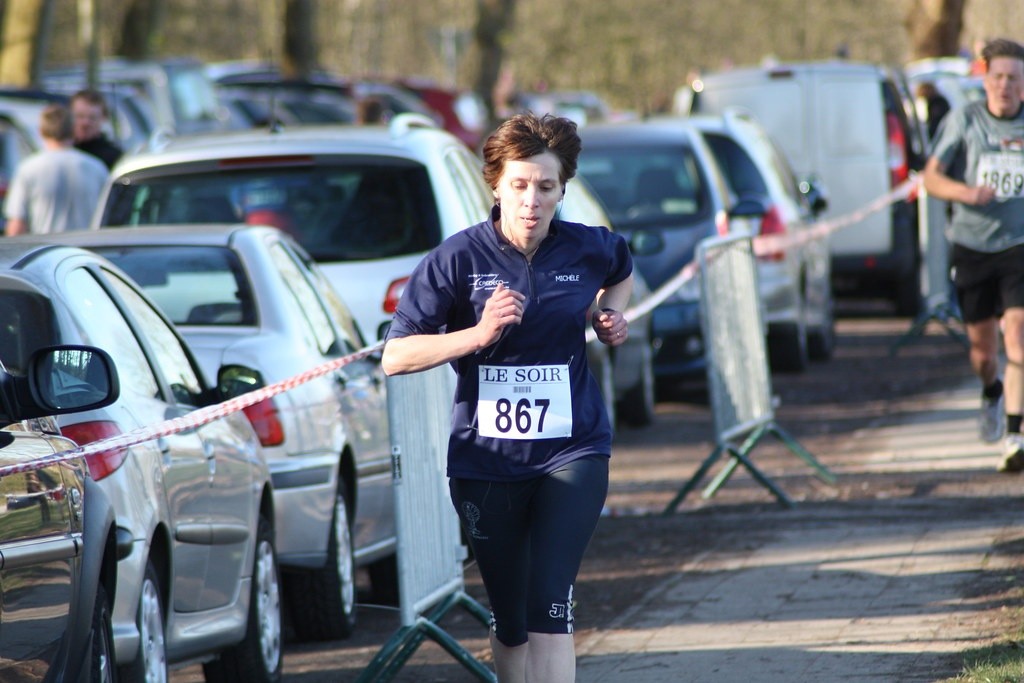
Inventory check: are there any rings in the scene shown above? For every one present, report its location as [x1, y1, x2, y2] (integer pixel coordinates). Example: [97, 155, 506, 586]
[616, 332, 620, 338]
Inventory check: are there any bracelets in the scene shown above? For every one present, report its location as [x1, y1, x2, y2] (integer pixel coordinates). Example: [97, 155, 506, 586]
[602, 308, 614, 312]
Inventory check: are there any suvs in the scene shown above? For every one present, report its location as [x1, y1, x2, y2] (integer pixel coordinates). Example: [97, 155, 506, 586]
[86, 110, 498, 405]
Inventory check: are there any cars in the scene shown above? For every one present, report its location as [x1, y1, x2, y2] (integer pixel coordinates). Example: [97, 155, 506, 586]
[1, 237, 287, 683]
[1, 219, 400, 642]
[0, 50, 989, 431]
[1, 345, 121, 683]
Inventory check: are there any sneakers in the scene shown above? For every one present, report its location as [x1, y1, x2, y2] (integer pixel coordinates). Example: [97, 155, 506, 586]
[995, 432, 1024, 473]
[979, 378, 1006, 442]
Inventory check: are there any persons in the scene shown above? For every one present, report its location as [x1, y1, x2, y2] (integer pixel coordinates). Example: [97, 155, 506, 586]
[925, 38, 1024, 472]
[2, 107, 109, 237]
[916, 82, 949, 139]
[380, 113, 634, 683]
[72, 89, 133, 226]
